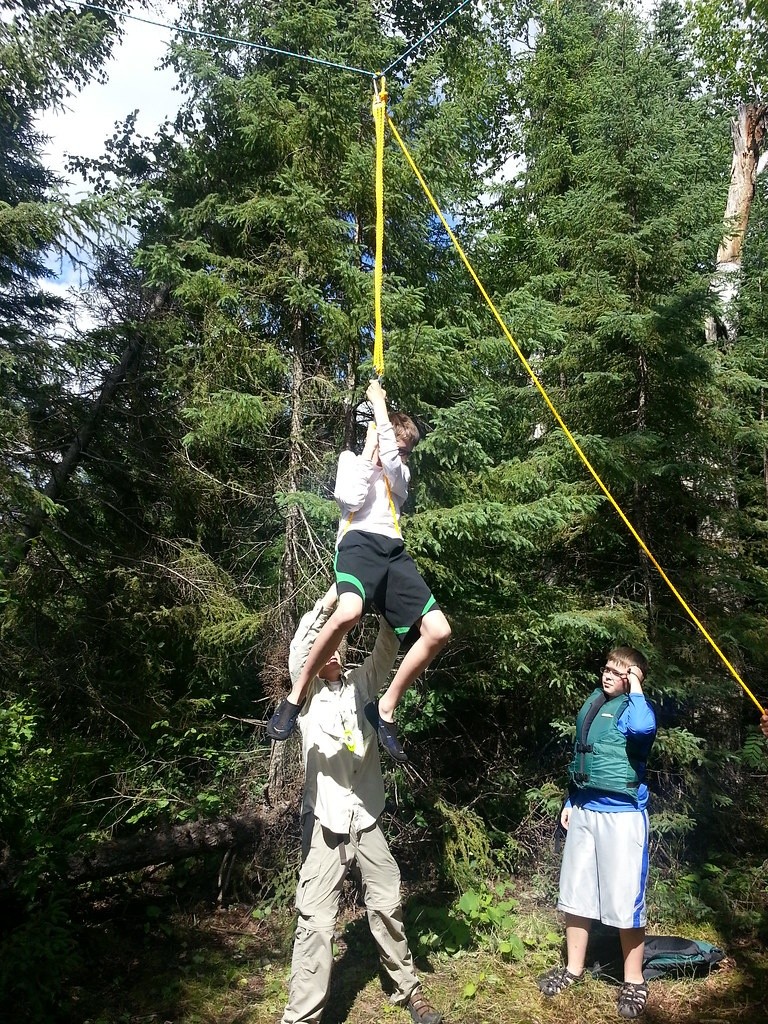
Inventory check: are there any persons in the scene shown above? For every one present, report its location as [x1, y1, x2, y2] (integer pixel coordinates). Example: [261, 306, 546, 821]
[263, 376, 451, 767]
[536, 646, 659, 1019]
[278, 578, 446, 1024]
[759, 709, 768, 738]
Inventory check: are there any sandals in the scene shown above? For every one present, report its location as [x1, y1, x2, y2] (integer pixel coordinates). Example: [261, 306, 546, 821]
[407, 985, 441, 1024]
[617, 980, 649, 1018]
[540, 969, 588, 996]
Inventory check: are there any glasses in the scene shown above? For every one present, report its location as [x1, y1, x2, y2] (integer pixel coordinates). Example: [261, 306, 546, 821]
[599, 666, 628, 681]
[398, 448, 413, 458]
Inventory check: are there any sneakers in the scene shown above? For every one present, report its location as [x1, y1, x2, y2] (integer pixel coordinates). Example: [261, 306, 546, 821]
[363, 700, 408, 763]
[267, 696, 307, 741]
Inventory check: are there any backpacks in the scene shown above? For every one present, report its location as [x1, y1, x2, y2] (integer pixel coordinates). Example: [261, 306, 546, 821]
[595, 935, 723, 984]
